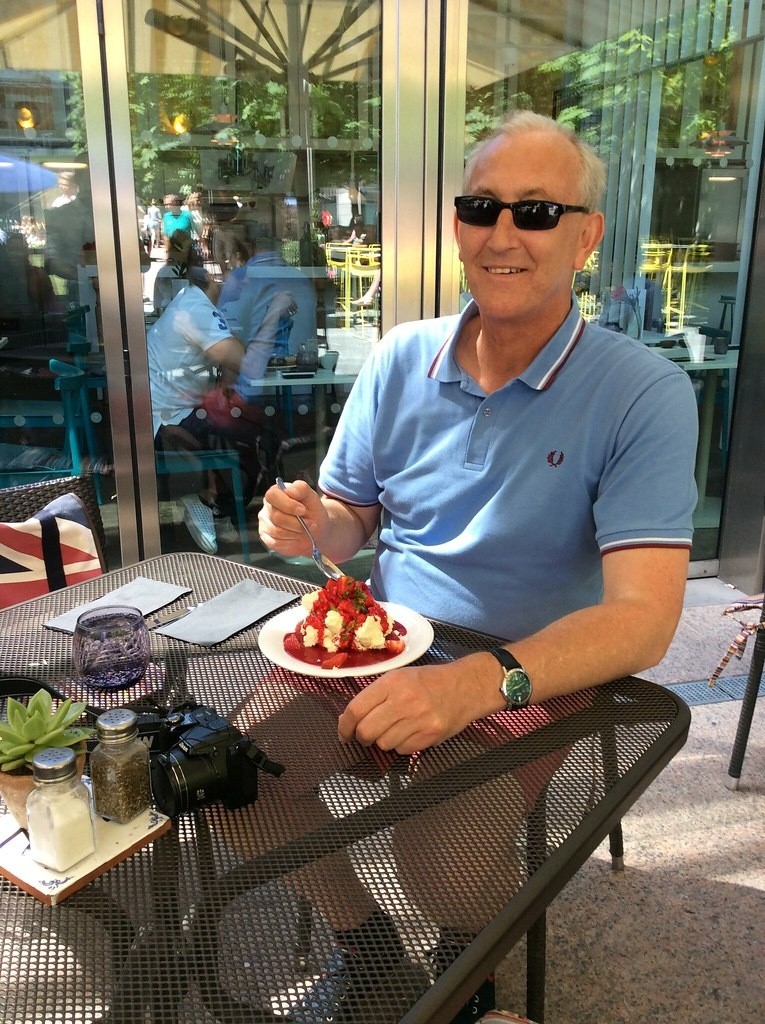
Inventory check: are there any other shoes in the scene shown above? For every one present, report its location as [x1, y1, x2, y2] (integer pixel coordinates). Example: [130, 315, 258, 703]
[424, 929, 497, 1024]
[287, 951, 412, 1024]
[215, 517, 240, 543]
[176, 494, 218, 555]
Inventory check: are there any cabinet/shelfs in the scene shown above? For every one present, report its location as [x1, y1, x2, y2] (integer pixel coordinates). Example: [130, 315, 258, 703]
[0, 0, 380, 154]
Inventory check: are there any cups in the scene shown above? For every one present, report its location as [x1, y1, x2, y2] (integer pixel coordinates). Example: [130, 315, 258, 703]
[72, 605, 150, 692]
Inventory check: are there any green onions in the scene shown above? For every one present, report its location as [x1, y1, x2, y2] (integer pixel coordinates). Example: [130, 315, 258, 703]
[330, 578, 369, 639]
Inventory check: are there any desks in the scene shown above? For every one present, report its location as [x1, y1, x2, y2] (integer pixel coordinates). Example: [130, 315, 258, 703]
[0, 552, 693, 1024]
[236, 369, 358, 494]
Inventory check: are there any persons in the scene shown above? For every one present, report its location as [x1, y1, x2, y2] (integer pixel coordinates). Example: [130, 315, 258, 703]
[207, 110, 699, 1024]
[0, 164, 209, 357]
[146, 202, 320, 556]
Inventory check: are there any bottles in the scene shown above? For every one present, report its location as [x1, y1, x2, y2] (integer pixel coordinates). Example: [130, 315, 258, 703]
[25, 746, 96, 872]
[89, 709, 152, 824]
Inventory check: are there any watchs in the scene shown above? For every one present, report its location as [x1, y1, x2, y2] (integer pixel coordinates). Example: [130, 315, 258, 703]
[487, 647, 533, 710]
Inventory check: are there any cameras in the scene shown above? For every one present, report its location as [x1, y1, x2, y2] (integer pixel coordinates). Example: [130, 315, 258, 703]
[148, 702, 258, 818]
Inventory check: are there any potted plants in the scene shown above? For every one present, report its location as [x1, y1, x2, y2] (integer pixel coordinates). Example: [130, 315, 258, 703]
[0, 689, 100, 829]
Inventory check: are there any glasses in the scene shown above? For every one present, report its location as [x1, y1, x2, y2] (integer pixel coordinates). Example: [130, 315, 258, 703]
[454, 196, 591, 231]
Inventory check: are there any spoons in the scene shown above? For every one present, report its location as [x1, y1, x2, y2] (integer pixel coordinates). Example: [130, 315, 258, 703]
[276, 477, 346, 581]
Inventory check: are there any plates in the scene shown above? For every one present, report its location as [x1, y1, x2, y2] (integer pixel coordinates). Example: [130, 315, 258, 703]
[257, 600, 434, 678]
[267, 365, 297, 368]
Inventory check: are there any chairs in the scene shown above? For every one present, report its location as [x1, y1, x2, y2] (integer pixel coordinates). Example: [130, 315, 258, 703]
[0, 374, 252, 574]
[294, 688, 624, 1023]
